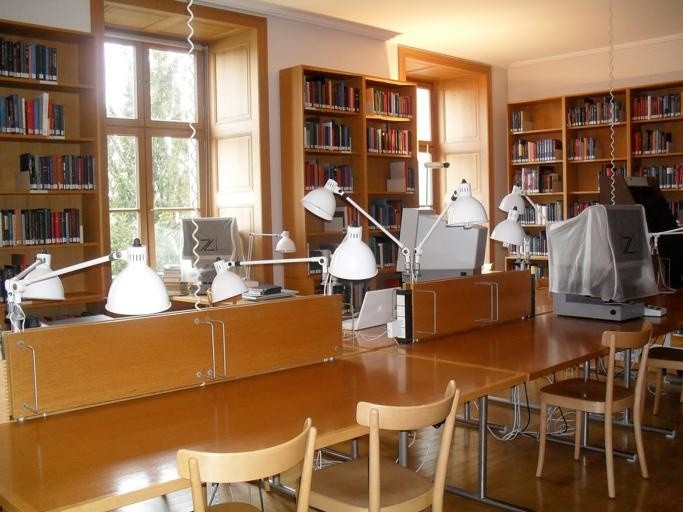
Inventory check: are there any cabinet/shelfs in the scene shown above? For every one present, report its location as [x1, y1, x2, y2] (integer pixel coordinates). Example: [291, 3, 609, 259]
[1, 21, 110, 317]
[507, 97, 565, 263]
[279, 64, 366, 295]
[629, 85, 682, 261]
[363, 76, 417, 278]
[566, 87, 632, 262]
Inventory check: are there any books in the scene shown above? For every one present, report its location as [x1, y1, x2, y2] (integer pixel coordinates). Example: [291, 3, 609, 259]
[368, 86, 412, 118]
[566, 96, 625, 127]
[242, 286, 294, 302]
[306, 243, 337, 274]
[597, 163, 627, 191]
[0, 253, 30, 303]
[633, 125, 672, 155]
[368, 196, 404, 228]
[511, 137, 557, 163]
[303, 78, 359, 112]
[303, 120, 352, 151]
[631, 93, 682, 120]
[0, 92, 66, 139]
[511, 229, 548, 255]
[0, 37, 59, 81]
[509, 260, 543, 279]
[369, 235, 398, 268]
[633, 163, 683, 189]
[157, 263, 188, 296]
[0, 206, 84, 247]
[568, 136, 595, 161]
[517, 200, 562, 224]
[669, 199, 683, 226]
[305, 160, 354, 192]
[508, 109, 531, 133]
[16, 153, 97, 191]
[570, 198, 600, 218]
[323, 206, 361, 231]
[367, 127, 411, 155]
[513, 166, 562, 193]
[386, 160, 414, 193]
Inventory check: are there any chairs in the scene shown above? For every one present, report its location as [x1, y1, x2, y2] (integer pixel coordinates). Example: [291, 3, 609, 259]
[175, 419, 317, 510]
[536, 322, 654, 497]
[638, 346, 683, 425]
[295, 378, 461, 512]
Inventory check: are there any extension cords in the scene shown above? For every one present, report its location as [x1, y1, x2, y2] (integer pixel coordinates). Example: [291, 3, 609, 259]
[644, 303, 668, 316]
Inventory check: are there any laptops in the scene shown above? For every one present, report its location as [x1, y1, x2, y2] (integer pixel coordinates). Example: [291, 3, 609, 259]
[342, 288, 401, 331]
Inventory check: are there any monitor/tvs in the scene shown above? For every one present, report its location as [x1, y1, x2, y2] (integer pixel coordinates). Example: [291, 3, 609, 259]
[545, 203, 659, 273]
[395, 207, 487, 282]
[181, 219, 236, 267]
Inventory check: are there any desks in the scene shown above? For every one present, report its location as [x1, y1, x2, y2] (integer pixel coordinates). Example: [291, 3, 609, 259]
[378, 290, 682, 511]
[1, 348, 531, 512]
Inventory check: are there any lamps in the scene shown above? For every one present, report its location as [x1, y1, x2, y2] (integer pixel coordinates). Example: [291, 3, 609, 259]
[324, 224, 378, 313]
[206, 257, 331, 303]
[7, 242, 171, 329]
[302, 180, 414, 283]
[7, 252, 65, 333]
[492, 207, 541, 249]
[243, 232, 296, 290]
[417, 180, 493, 273]
[500, 181, 553, 220]
